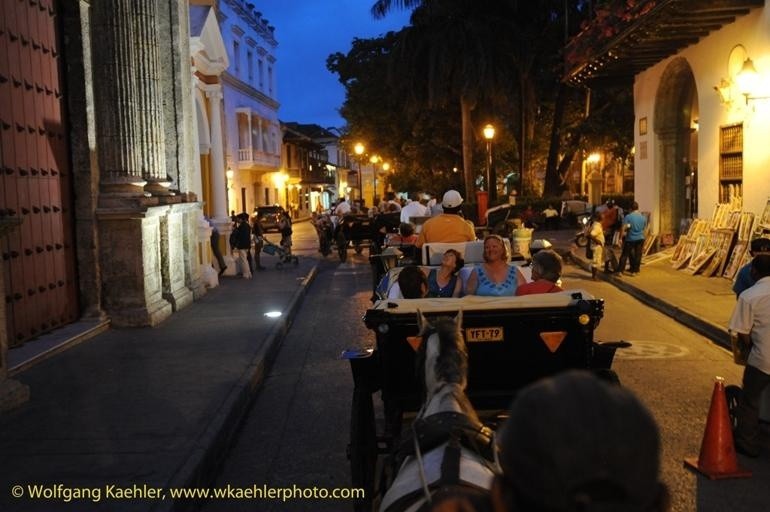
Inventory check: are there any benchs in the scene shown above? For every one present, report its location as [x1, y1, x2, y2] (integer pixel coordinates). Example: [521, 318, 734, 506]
[365, 227, 606, 430]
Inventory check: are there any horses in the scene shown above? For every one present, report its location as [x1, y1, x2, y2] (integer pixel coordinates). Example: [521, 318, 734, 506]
[380, 307, 502, 512]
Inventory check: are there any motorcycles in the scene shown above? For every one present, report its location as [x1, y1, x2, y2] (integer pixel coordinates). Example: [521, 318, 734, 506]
[575, 210, 600, 247]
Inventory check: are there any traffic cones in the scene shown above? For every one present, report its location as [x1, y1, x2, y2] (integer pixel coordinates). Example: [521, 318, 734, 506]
[682, 374, 756, 483]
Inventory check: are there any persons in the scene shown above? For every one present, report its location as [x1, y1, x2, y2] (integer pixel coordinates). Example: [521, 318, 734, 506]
[426, 249, 466, 298]
[492, 203, 561, 269]
[204, 213, 227, 276]
[515, 248, 565, 296]
[308, 189, 445, 255]
[387, 263, 429, 298]
[230, 210, 294, 280]
[387, 221, 418, 246]
[417, 189, 479, 242]
[585, 196, 648, 281]
[465, 233, 527, 296]
[725, 253, 770, 458]
[731, 237, 770, 301]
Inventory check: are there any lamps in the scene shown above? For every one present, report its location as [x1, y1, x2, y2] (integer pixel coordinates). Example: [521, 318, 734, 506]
[736, 57, 768, 106]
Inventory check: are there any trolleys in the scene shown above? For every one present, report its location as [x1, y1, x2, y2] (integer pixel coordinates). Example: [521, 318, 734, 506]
[258, 234, 299, 269]
[722, 331, 770, 454]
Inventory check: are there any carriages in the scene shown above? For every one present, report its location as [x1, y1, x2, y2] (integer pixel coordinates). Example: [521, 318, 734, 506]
[312, 200, 635, 512]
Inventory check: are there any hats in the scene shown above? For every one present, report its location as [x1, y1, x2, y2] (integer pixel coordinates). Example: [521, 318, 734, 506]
[442, 190, 464, 208]
[751, 238, 770, 252]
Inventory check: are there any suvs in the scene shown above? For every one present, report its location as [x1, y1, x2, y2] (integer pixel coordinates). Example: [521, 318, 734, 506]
[249, 204, 292, 233]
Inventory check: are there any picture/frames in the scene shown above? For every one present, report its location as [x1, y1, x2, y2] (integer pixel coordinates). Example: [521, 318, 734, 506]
[671, 200, 770, 279]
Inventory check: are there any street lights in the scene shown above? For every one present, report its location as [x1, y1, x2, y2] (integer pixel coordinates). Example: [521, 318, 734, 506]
[353, 142, 365, 200]
[371, 155, 379, 200]
[382, 160, 390, 196]
[484, 122, 498, 210]
